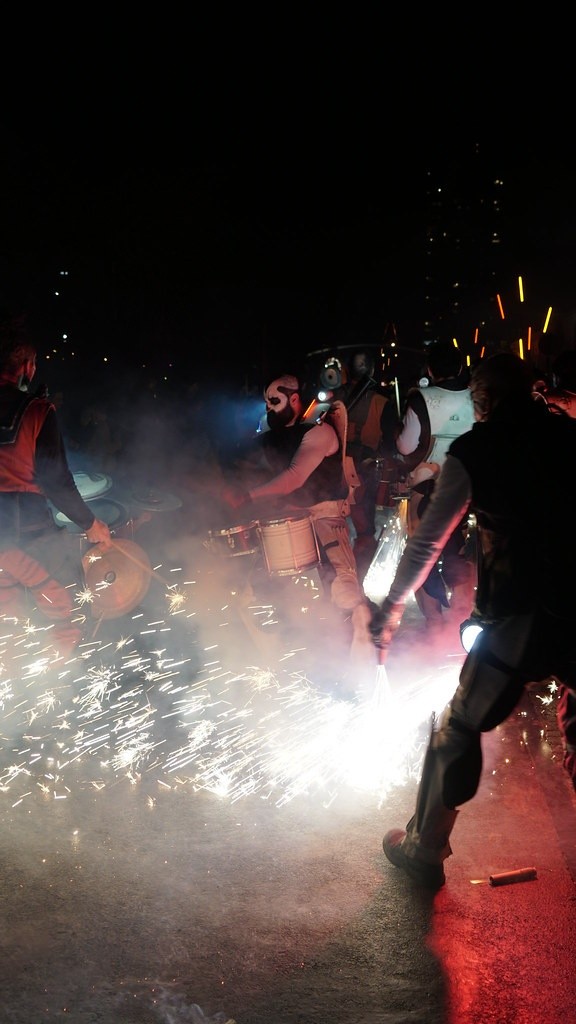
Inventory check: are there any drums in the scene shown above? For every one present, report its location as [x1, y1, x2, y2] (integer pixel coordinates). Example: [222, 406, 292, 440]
[50, 498, 136, 562]
[203, 520, 262, 560]
[260, 508, 322, 579]
[68, 469, 115, 503]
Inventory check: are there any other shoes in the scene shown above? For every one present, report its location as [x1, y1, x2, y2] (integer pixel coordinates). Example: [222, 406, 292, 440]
[382, 829, 446, 888]
[353, 536, 377, 560]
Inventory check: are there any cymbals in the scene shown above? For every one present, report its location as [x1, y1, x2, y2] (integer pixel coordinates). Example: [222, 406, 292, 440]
[121, 487, 183, 514]
[75, 537, 152, 621]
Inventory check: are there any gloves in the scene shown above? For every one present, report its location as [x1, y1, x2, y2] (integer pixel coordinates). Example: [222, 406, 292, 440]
[368, 598, 407, 647]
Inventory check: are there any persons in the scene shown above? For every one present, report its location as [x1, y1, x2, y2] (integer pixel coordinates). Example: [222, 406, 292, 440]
[0, 342, 576, 748]
[369, 356, 576, 886]
